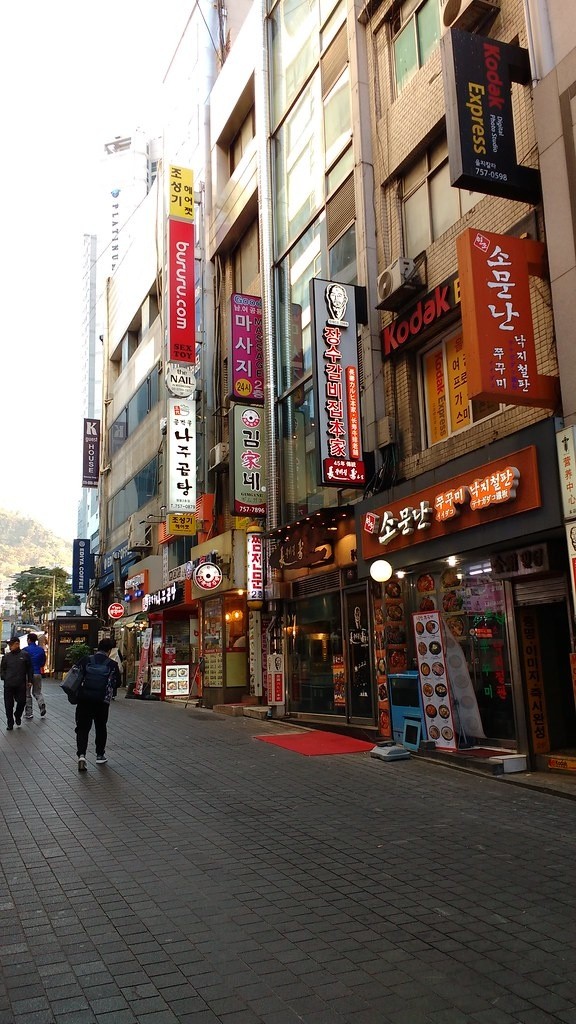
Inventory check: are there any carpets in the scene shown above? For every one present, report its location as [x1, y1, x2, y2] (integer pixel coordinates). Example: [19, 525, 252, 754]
[256, 731, 376, 756]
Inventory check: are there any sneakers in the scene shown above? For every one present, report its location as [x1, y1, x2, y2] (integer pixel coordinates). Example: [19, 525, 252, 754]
[96, 754, 108, 763]
[78, 755, 87, 771]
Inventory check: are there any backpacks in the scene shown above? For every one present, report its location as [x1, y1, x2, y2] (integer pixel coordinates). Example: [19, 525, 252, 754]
[81, 655, 112, 701]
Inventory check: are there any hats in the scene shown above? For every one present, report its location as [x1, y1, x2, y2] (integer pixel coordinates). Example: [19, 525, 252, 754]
[6, 637, 20, 644]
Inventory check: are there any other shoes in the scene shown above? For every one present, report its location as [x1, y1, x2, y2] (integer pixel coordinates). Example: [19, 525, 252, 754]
[7, 724, 13, 730]
[39, 702, 46, 716]
[23, 713, 33, 719]
[14, 711, 21, 725]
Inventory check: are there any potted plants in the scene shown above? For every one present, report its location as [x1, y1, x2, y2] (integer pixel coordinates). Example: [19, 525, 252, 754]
[63, 640, 90, 680]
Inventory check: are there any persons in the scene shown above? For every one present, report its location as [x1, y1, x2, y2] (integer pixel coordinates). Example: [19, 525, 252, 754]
[0, 637, 35, 729]
[20, 633, 47, 719]
[74, 638, 125, 771]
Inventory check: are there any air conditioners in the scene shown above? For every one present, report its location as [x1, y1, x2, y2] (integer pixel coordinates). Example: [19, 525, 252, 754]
[376, 257, 419, 306]
[209, 443, 229, 470]
[439, 0, 473, 37]
[128, 512, 147, 550]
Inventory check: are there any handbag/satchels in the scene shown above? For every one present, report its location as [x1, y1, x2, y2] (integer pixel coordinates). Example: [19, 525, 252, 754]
[104, 662, 114, 704]
[59, 655, 88, 704]
